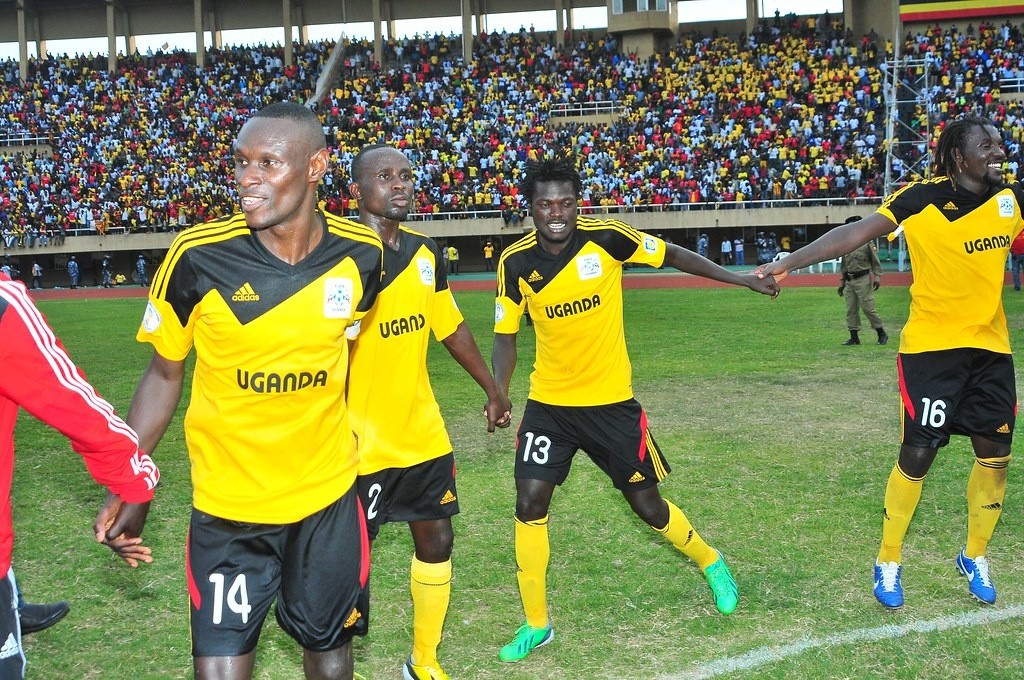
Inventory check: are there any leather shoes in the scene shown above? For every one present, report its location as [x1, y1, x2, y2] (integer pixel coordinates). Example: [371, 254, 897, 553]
[17, 591, 70, 637]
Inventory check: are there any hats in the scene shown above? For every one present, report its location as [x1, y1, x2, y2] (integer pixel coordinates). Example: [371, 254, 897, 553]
[758, 232, 764, 235]
[138, 255, 143, 258]
[845, 215, 863, 224]
[702, 234, 707, 237]
[487, 242, 491, 244]
[104, 256, 110, 258]
[72, 256, 75, 260]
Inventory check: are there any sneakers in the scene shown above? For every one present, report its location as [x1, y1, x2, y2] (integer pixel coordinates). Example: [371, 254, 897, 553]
[702, 549, 739, 616]
[957, 547, 996, 606]
[403, 652, 450, 680]
[874, 556, 904, 609]
[500, 618, 554, 662]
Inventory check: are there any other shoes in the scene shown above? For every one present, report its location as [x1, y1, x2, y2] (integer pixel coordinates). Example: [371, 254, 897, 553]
[141, 283, 144, 287]
[110, 284, 115, 288]
[71, 284, 78, 290]
[841, 337, 860, 345]
[104, 283, 108, 288]
[146, 283, 151, 286]
[878, 333, 888, 345]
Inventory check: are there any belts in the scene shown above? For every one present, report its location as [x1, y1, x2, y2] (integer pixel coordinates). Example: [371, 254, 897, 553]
[845, 269, 869, 281]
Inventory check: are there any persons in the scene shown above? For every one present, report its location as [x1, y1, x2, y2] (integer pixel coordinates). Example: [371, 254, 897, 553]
[328, 145, 511, 680]
[836, 215, 889, 347]
[752, 116, 1024, 612]
[91, 104, 384, 679]
[484, 150, 781, 663]
[0, 271, 161, 680]
[0, 9, 1023, 290]
